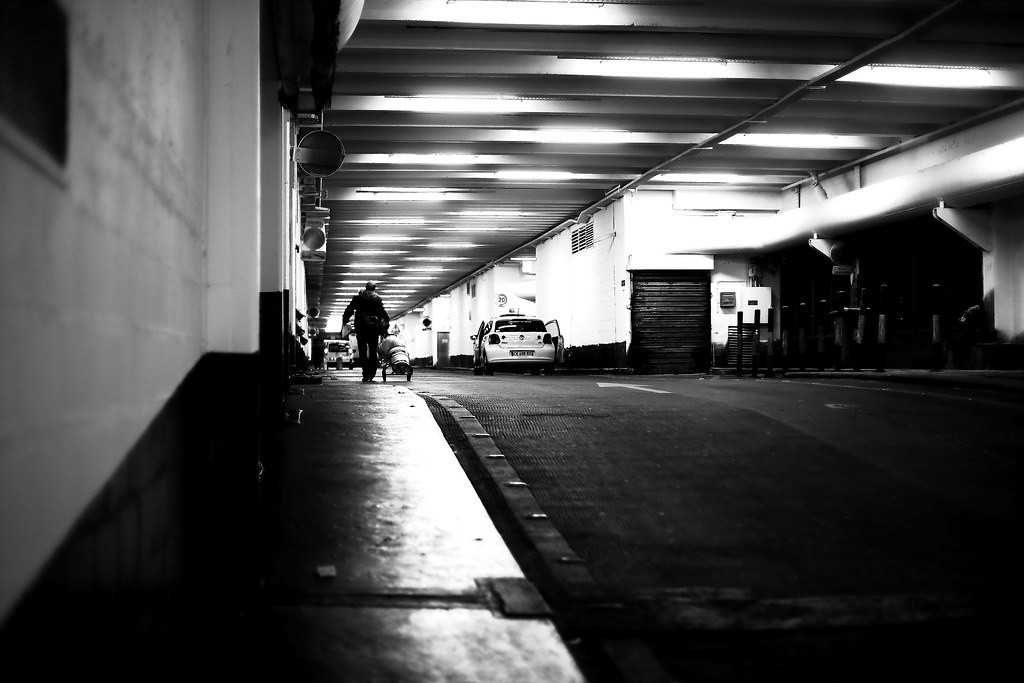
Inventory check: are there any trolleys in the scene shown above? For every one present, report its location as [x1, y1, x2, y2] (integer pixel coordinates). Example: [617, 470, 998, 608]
[379, 333, 414, 381]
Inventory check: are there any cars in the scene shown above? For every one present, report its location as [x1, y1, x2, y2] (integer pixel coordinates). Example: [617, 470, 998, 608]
[470, 314, 564, 377]
[323, 340, 357, 370]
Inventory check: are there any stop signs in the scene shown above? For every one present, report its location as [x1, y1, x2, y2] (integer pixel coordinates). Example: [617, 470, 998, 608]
[295, 129, 346, 178]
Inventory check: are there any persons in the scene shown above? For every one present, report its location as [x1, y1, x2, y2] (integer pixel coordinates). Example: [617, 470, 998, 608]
[342, 281, 390, 382]
[313, 334, 327, 370]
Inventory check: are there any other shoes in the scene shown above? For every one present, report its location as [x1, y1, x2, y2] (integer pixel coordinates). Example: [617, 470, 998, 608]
[361, 379, 376, 384]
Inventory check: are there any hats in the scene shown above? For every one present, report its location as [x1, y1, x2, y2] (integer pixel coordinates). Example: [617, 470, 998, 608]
[365, 282, 376, 287]
